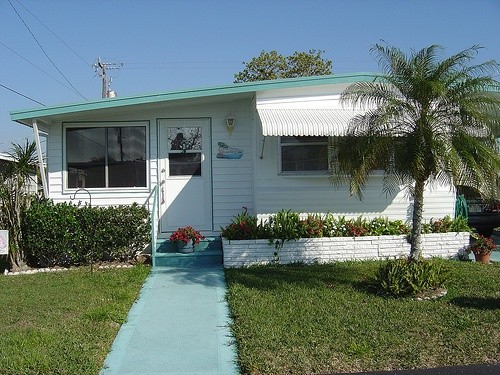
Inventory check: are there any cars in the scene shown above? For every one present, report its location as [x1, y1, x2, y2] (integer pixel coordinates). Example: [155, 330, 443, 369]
[455, 184, 500, 239]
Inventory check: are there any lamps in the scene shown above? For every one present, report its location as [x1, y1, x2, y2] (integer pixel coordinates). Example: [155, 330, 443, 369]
[226, 112, 235, 135]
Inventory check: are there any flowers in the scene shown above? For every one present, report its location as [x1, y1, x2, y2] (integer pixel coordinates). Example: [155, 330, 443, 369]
[170, 226, 204, 245]
[471, 235, 495, 254]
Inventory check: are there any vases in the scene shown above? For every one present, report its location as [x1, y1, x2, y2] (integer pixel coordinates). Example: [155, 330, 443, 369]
[177, 238, 194, 252]
[475, 251, 491, 264]
